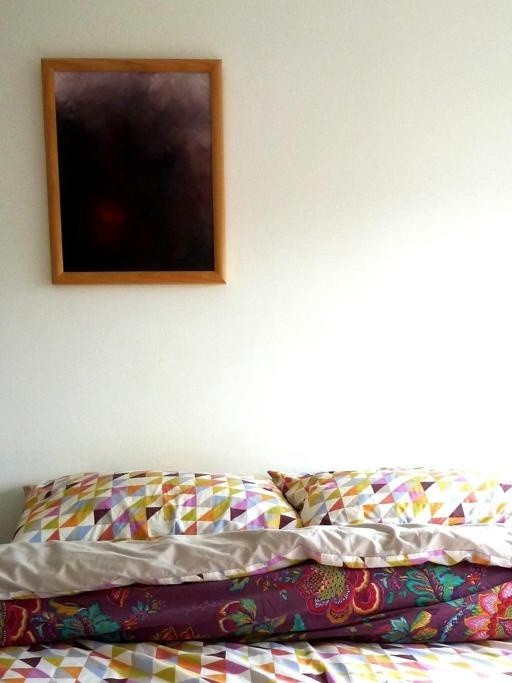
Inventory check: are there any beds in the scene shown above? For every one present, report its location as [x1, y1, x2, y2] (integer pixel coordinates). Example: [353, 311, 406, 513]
[0, 540, 512, 683]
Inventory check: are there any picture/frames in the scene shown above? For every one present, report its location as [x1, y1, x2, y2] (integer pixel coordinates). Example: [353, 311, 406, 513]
[40, 56, 228, 287]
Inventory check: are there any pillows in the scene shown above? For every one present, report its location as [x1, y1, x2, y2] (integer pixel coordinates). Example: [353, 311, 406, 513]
[267, 467, 512, 528]
[10, 468, 304, 545]
